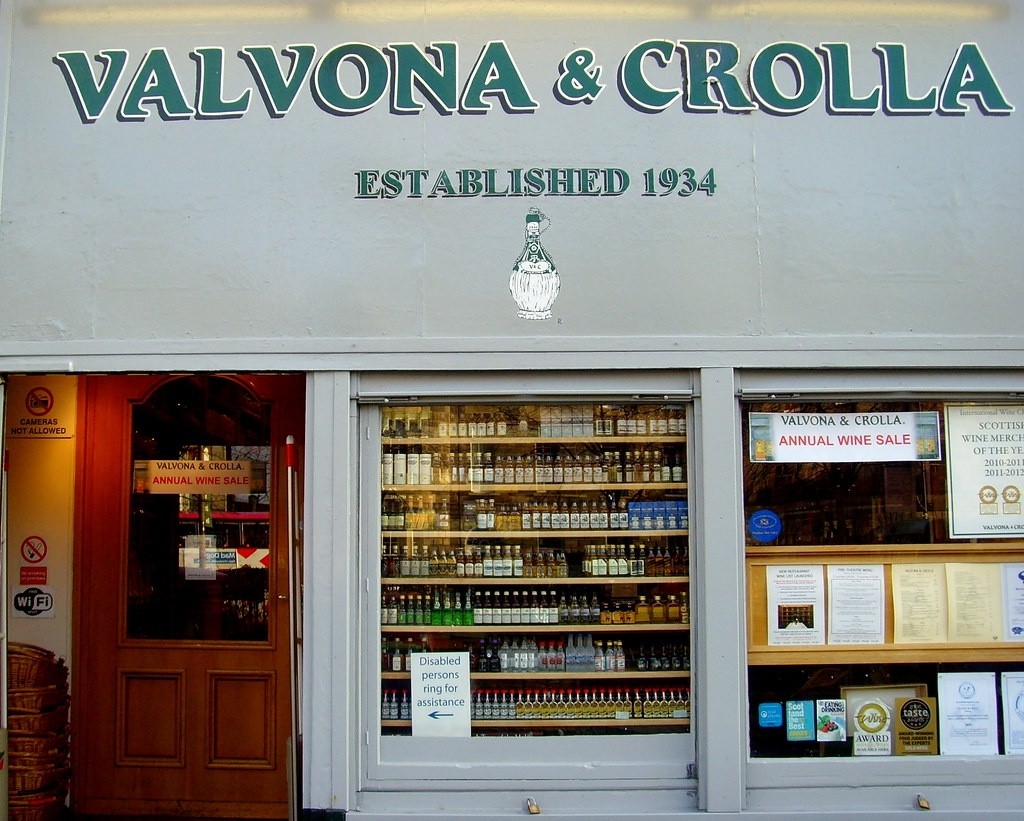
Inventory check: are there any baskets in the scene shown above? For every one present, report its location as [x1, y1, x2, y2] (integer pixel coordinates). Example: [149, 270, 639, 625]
[6, 641, 73, 821]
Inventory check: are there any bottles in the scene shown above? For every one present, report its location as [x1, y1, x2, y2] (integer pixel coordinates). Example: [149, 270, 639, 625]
[382, 450, 684, 484]
[382, 544, 688, 578]
[381, 406, 687, 436]
[381, 493, 630, 530]
[381, 585, 690, 625]
[382, 634, 690, 671]
[380, 688, 690, 719]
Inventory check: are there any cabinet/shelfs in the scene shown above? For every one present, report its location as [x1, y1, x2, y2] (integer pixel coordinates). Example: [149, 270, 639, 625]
[379, 403, 692, 726]
